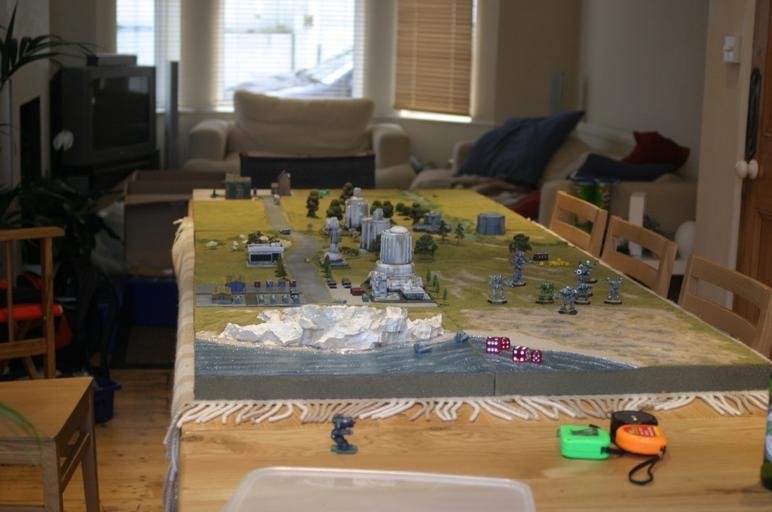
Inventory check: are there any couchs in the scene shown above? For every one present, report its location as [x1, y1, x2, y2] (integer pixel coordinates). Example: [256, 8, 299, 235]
[408, 119, 696, 236]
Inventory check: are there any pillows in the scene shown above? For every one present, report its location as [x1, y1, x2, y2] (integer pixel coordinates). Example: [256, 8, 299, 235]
[452, 109, 587, 190]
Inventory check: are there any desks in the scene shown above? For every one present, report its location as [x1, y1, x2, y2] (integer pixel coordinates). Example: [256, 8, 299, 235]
[177, 184, 772, 512]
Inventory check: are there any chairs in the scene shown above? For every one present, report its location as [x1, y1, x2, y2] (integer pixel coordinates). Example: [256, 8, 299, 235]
[677, 253, 772, 359]
[599, 215, 678, 298]
[0, 225, 66, 383]
[184, 89, 416, 189]
[549, 190, 609, 260]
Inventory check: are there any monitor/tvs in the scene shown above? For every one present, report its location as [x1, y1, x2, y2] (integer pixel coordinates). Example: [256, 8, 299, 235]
[49, 66, 156, 170]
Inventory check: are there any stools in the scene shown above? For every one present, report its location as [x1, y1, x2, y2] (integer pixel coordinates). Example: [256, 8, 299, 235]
[0, 377, 100, 512]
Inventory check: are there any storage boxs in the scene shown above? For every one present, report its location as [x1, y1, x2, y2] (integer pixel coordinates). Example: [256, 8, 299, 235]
[113, 169, 227, 279]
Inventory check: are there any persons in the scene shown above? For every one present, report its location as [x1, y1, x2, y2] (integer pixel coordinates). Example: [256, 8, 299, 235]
[558, 260, 623, 311]
[487, 249, 528, 301]
[305, 182, 423, 226]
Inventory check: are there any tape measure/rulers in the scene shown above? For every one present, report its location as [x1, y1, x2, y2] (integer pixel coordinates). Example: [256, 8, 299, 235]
[557, 410, 667, 484]
